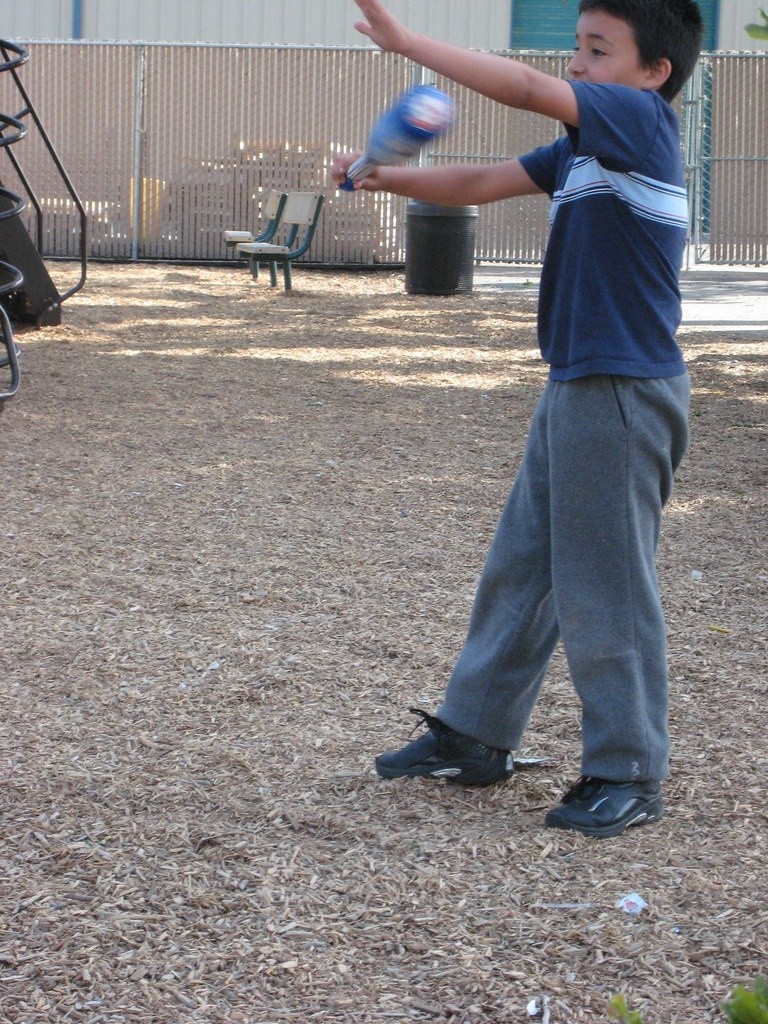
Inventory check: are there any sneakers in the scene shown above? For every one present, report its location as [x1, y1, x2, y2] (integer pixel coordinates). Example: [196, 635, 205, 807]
[376, 707, 515, 786]
[543, 775, 664, 836]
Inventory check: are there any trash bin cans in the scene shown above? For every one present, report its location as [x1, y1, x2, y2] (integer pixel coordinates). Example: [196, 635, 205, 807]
[403, 198, 478, 295]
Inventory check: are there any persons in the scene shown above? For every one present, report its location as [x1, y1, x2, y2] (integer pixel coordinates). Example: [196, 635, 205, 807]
[331, 0, 702, 840]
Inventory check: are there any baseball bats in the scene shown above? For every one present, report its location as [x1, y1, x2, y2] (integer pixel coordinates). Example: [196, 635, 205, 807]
[337, 84, 457, 193]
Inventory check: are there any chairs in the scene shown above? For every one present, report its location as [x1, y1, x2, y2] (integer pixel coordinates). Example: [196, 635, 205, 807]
[237, 192, 325, 291]
[223, 189, 287, 280]
